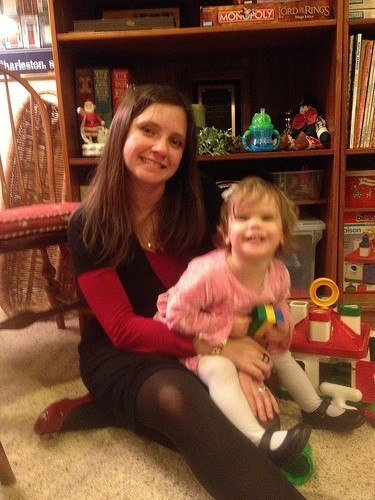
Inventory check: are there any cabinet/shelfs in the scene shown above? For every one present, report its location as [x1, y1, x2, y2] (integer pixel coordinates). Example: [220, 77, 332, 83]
[47, 0, 375, 326]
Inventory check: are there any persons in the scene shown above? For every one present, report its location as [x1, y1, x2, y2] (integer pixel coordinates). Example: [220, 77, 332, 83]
[158, 176, 365, 461]
[33, 84, 309, 500]
[77, 99, 105, 144]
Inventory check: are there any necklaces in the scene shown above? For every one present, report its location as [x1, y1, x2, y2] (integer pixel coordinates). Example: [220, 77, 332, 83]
[141, 229, 152, 248]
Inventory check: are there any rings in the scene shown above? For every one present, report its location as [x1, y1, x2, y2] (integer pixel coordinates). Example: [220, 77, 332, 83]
[258, 385, 267, 392]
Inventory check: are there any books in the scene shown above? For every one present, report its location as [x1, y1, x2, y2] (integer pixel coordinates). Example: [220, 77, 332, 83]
[75, 67, 131, 157]
[347, 33, 375, 148]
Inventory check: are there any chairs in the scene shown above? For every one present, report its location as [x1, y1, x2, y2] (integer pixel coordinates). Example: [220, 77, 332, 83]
[0, 65, 86, 484]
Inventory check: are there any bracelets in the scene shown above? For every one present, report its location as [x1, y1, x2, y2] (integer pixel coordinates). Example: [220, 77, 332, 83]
[211, 338, 228, 355]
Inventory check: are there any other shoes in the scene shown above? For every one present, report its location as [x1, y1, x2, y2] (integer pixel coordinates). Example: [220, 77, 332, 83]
[34, 393, 95, 435]
[260, 423, 312, 466]
[302, 399, 364, 430]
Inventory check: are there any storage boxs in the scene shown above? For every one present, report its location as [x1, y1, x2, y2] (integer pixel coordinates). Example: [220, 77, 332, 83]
[343, 169, 375, 294]
[269, 167, 325, 201]
[285, 211, 326, 287]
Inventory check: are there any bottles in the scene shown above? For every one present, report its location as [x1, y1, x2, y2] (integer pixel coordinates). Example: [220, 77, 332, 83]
[242, 108, 281, 152]
